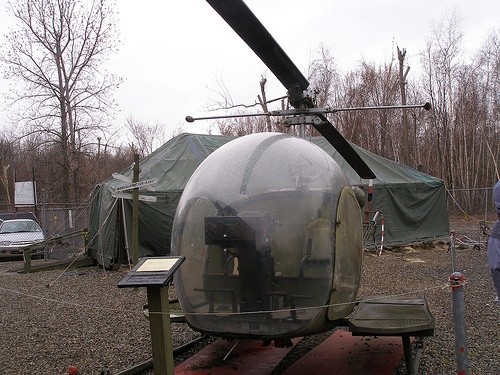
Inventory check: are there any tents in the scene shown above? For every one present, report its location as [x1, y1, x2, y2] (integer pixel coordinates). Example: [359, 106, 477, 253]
[86, 132, 450, 271]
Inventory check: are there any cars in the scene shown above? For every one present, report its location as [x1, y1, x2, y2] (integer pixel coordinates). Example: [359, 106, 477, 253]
[0, 211, 46, 262]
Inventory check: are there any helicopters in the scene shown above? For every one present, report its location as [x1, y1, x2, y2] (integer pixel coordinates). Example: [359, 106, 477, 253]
[138, 0, 438, 375]
